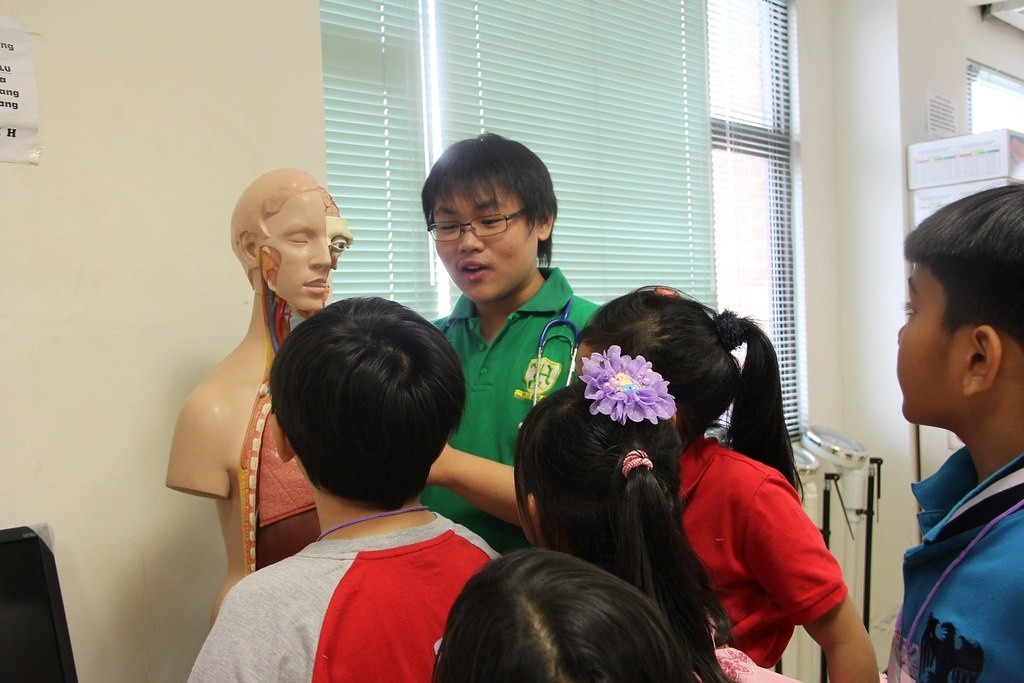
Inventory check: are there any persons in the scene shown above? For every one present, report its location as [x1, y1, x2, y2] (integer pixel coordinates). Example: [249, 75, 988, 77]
[885, 178, 1024, 683]
[412, 131, 608, 559]
[184, 296, 508, 683]
[166, 163, 356, 640]
[520, 379, 808, 683]
[427, 283, 884, 683]
[425, 548, 693, 682]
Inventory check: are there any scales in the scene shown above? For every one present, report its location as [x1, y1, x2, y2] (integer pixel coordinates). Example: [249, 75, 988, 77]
[790, 421, 884, 683]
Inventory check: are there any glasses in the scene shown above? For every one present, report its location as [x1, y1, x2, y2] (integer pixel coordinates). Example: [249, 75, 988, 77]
[426, 204, 535, 242]
